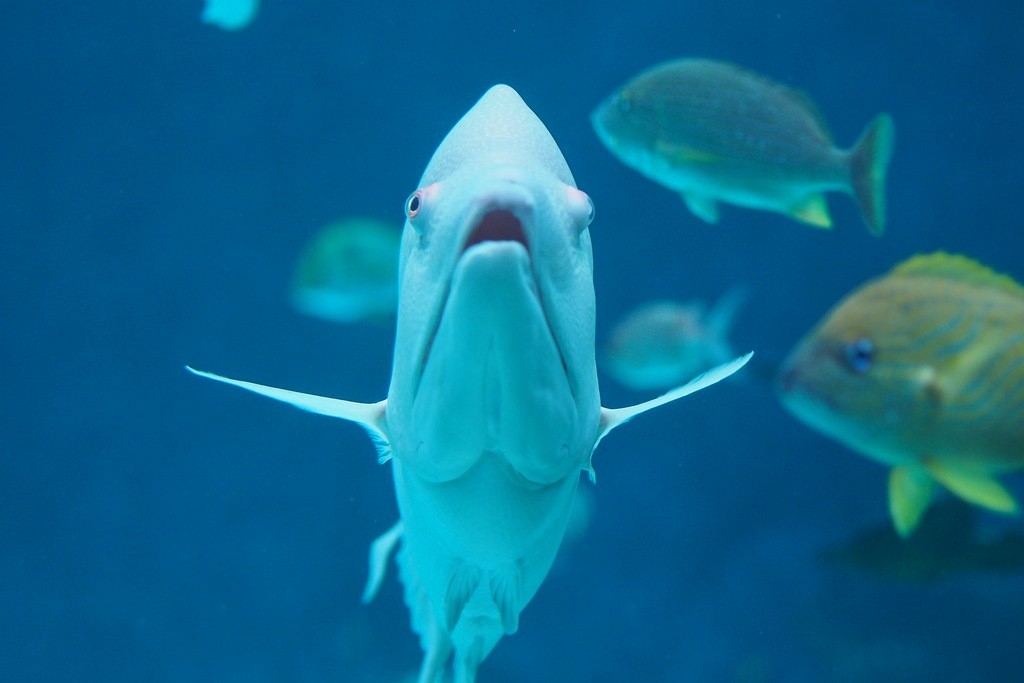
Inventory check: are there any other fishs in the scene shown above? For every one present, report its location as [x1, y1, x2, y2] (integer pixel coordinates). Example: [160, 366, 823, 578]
[184, 1, 1024, 683]
